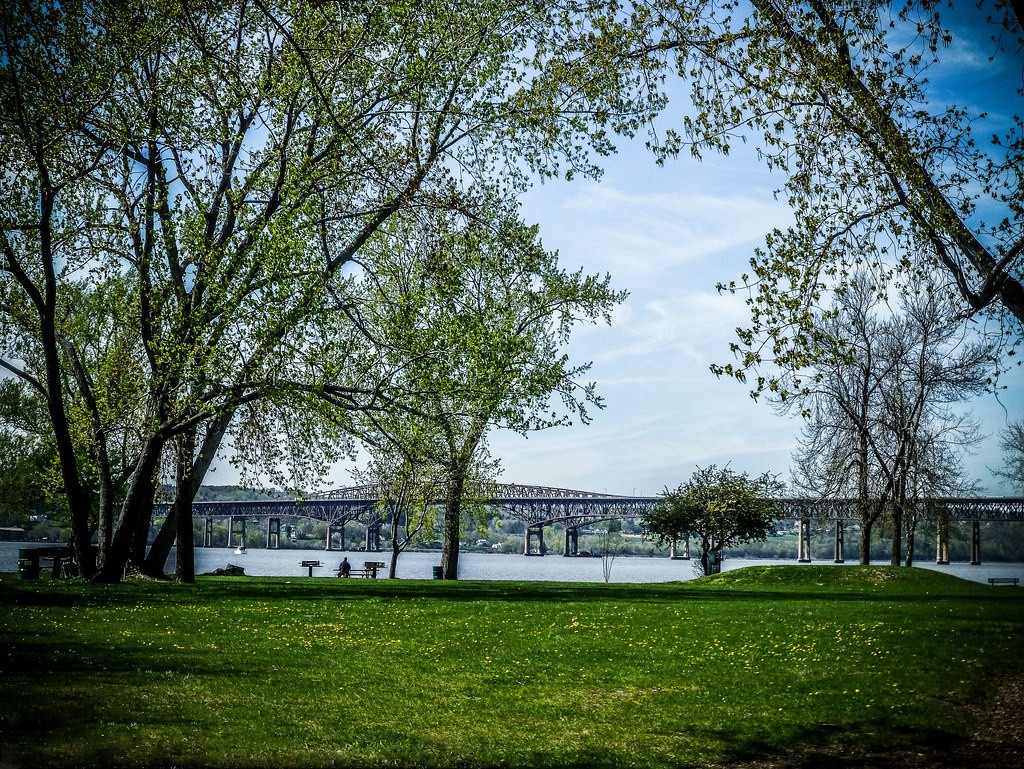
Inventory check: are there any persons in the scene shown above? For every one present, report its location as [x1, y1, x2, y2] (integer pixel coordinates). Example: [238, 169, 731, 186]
[338, 557, 351, 579]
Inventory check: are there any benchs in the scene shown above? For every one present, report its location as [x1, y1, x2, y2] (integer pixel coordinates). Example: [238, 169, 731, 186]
[988, 577, 1020, 592]
[38, 546, 98, 580]
[336, 574, 372, 579]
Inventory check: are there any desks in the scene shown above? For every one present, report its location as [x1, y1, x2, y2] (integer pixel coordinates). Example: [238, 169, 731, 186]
[333, 569, 379, 578]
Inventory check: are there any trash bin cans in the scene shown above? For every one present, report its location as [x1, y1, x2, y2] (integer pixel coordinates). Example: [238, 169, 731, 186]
[16, 548, 40, 579]
[432, 564, 444, 581]
